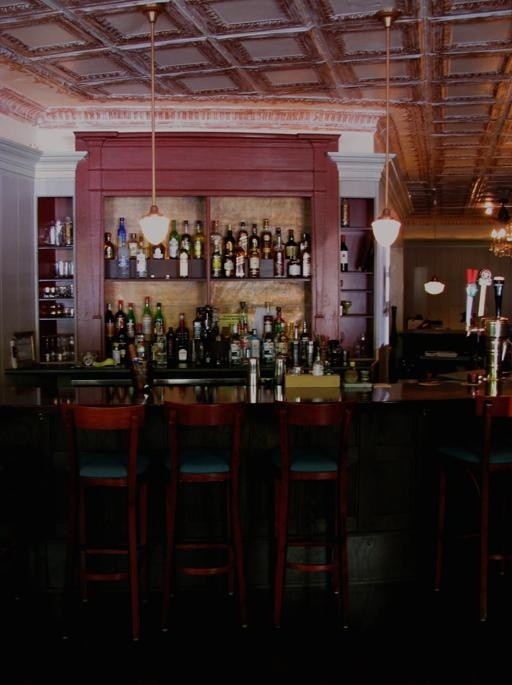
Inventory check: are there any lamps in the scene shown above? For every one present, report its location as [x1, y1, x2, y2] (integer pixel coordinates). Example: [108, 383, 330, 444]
[140, 4, 169, 246]
[370, 10, 402, 250]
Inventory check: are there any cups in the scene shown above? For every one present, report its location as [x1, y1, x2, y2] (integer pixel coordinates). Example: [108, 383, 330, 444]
[58, 286, 68, 297]
[467, 372, 479, 384]
[63, 307, 74, 318]
[53, 259, 73, 277]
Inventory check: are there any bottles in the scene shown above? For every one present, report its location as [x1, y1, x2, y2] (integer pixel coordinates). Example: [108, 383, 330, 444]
[102, 214, 312, 279]
[49, 216, 73, 247]
[312, 346, 369, 384]
[360, 335, 367, 358]
[103, 294, 345, 365]
[48, 303, 63, 317]
[247, 385, 259, 404]
[273, 385, 285, 403]
[245, 358, 261, 387]
[44, 287, 56, 299]
[272, 354, 288, 385]
[44, 333, 75, 362]
[340, 199, 352, 229]
[340, 235, 349, 273]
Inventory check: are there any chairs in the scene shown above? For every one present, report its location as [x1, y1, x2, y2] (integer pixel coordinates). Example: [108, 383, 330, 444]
[433, 395, 510, 623]
